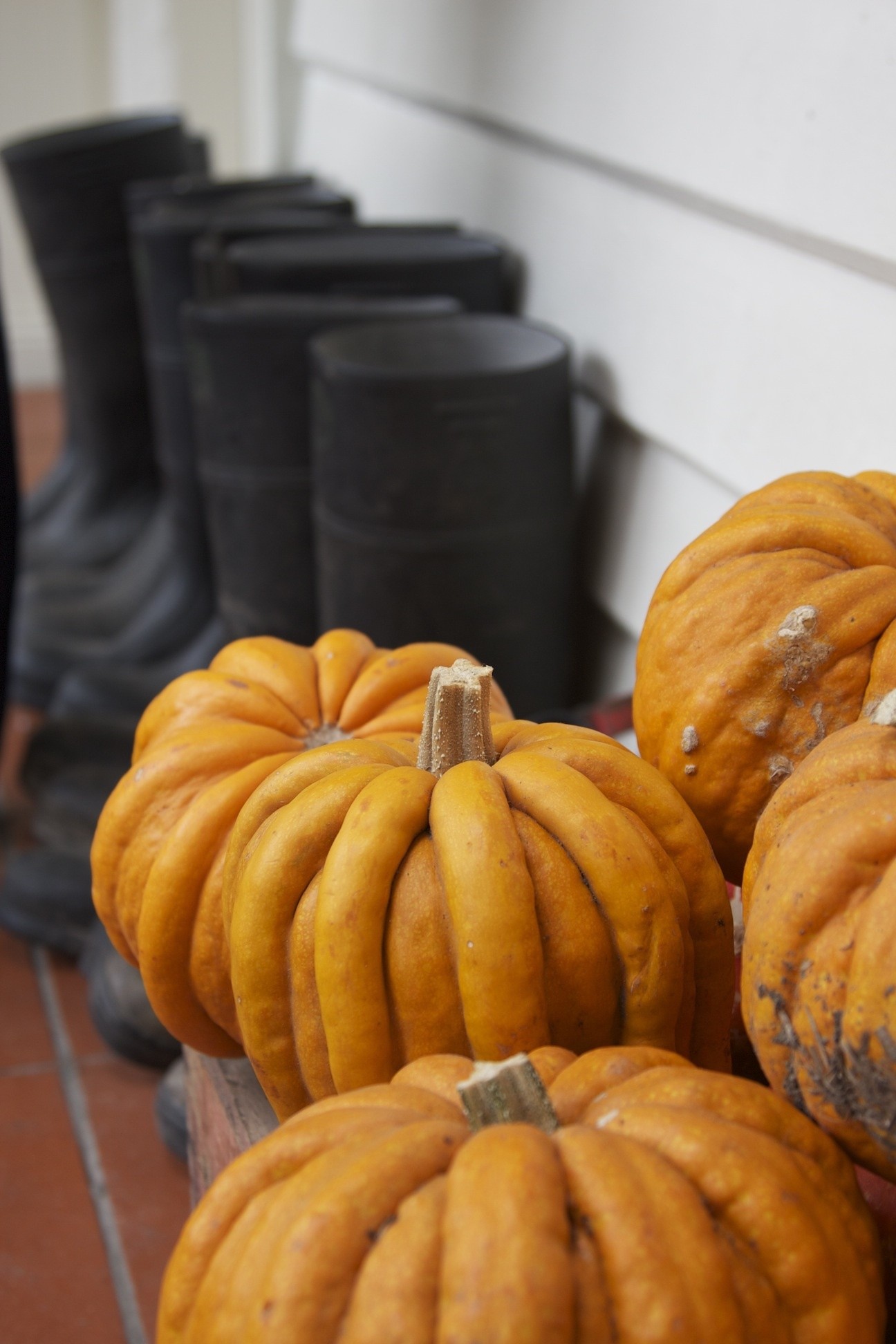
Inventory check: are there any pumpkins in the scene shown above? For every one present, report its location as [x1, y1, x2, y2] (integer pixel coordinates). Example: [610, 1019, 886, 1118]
[153, 1041, 888, 1344]
[740, 688, 896, 1177]
[632, 470, 896, 886]
[91, 627, 512, 1058]
[219, 655, 733, 1125]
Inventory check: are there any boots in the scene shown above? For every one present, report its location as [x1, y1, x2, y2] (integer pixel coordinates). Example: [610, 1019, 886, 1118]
[0, 122, 577, 1065]
